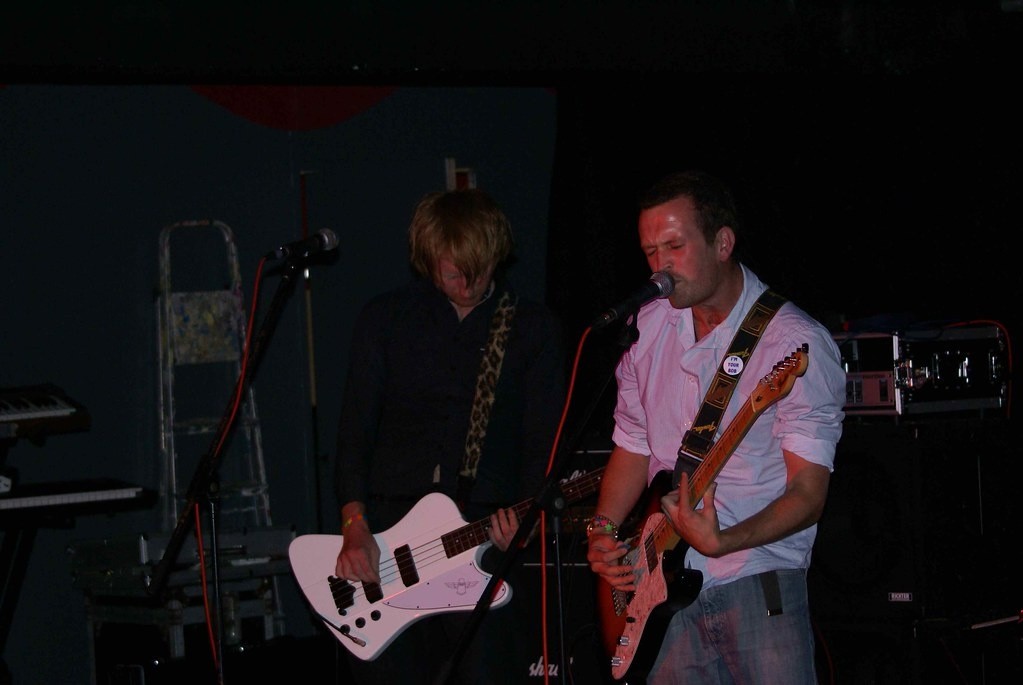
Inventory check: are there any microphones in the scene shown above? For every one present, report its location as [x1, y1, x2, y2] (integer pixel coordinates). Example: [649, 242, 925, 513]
[266, 227, 341, 260]
[592, 271, 676, 330]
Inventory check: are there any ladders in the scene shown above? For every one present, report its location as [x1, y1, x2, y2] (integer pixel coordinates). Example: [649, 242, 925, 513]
[156, 218, 288, 658]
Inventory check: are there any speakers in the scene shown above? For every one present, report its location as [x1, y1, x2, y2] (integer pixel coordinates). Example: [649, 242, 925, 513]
[514, 555, 601, 685]
[807, 417, 1023, 685]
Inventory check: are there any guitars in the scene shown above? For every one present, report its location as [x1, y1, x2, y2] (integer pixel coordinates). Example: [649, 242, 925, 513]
[599, 341, 810, 683]
[287, 460, 606, 663]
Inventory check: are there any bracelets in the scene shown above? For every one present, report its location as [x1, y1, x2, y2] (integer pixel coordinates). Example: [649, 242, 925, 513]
[586, 514, 618, 541]
[340, 513, 366, 527]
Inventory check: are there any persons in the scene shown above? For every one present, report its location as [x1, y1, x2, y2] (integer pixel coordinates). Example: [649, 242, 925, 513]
[332, 189, 586, 685]
[585, 174, 846, 685]
